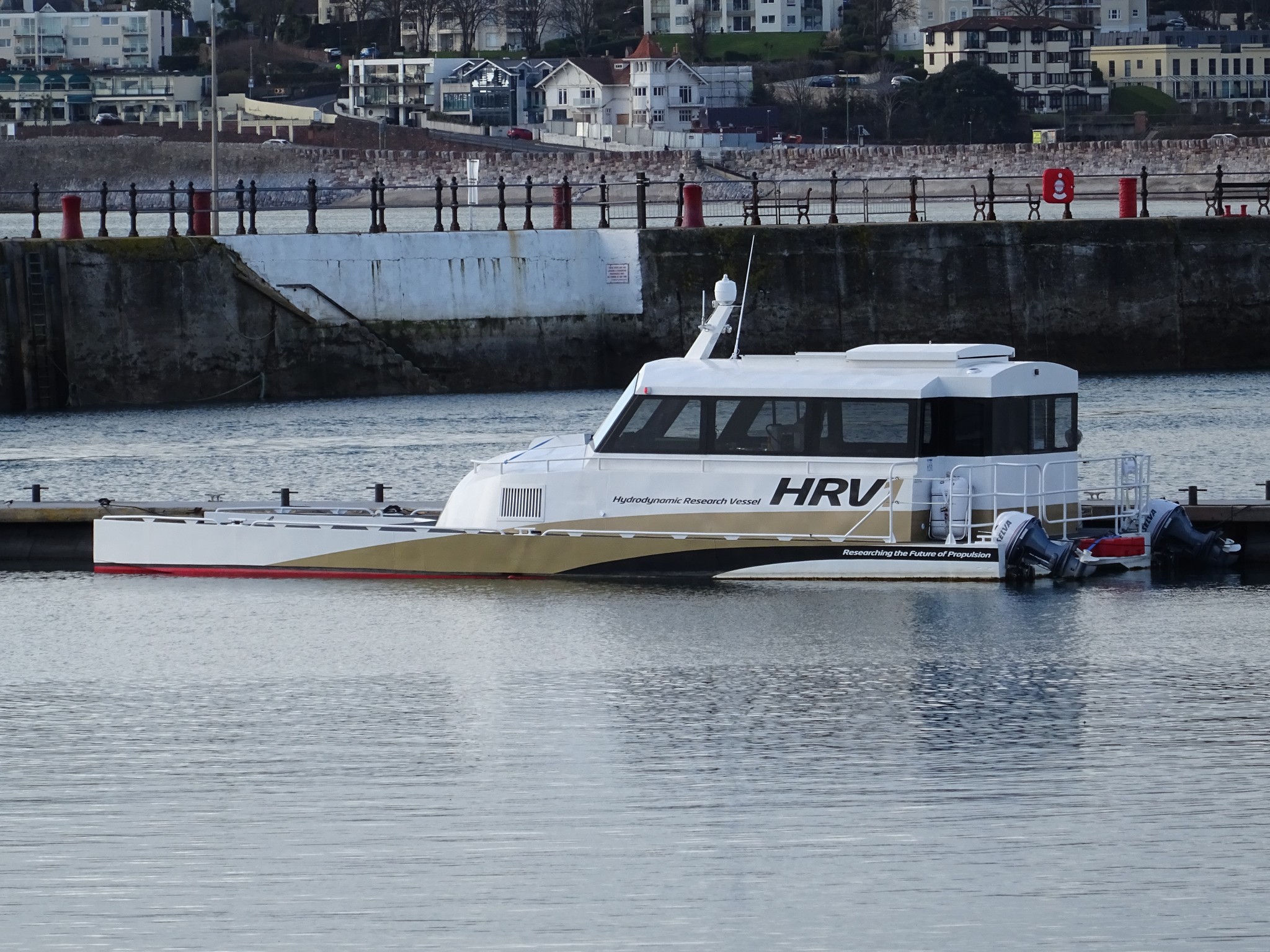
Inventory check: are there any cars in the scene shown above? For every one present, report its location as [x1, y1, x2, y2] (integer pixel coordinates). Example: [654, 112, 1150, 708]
[812, 75, 847, 87]
[1210, 134, 1238, 139]
[325, 48, 341, 56]
[262, 139, 292, 145]
[891, 75, 919, 89]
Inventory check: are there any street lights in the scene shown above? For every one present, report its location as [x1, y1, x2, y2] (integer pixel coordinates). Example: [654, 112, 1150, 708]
[336, 26, 342, 49]
[371, 42, 376, 59]
[839, 70, 849, 145]
[1057, 60, 1066, 142]
[822, 128, 827, 148]
[50, 79, 55, 137]
[969, 121, 973, 144]
[767, 110, 772, 143]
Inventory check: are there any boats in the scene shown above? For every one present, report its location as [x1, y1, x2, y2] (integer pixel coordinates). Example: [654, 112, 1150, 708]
[92, 233, 1242, 583]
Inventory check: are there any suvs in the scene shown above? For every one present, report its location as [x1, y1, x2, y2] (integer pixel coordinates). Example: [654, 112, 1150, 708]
[507, 127, 533, 140]
[94, 113, 123, 125]
[359, 48, 380, 59]
